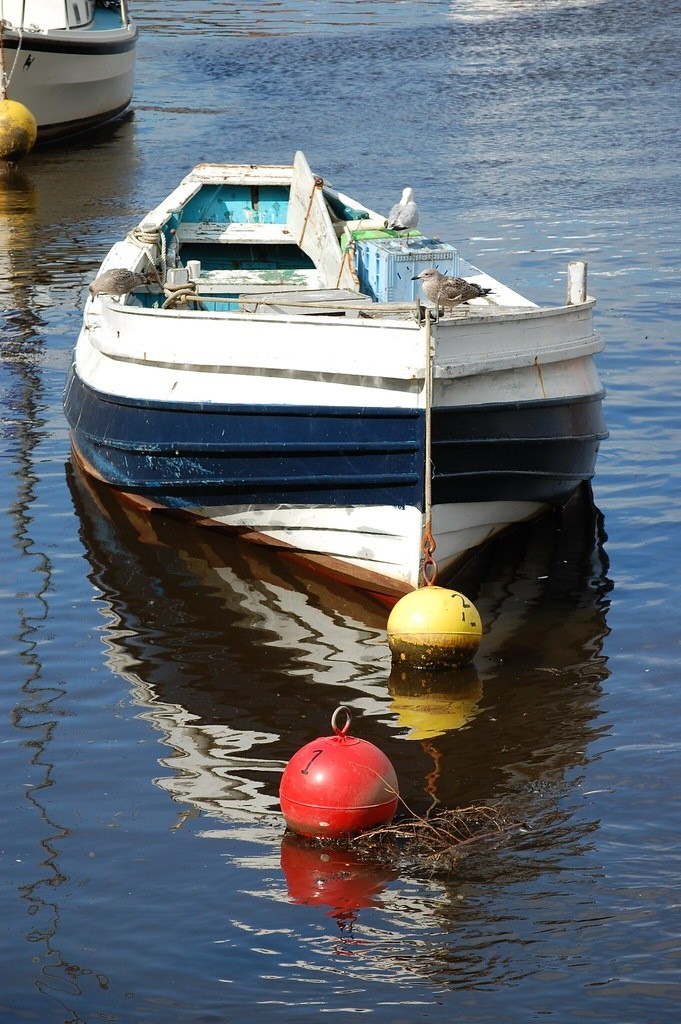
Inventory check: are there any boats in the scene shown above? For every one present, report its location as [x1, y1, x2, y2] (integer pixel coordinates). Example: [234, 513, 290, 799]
[63, 149, 609, 671]
[0, 0, 139, 166]
[63, 459, 620, 923]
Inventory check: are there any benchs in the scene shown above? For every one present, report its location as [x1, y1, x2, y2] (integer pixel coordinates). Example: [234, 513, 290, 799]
[133, 267, 329, 293]
[173, 221, 296, 245]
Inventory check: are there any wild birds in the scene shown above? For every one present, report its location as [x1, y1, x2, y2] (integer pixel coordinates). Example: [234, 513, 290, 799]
[89, 268, 151, 304]
[387, 186, 421, 245]
[411, 268, 495, 314]
